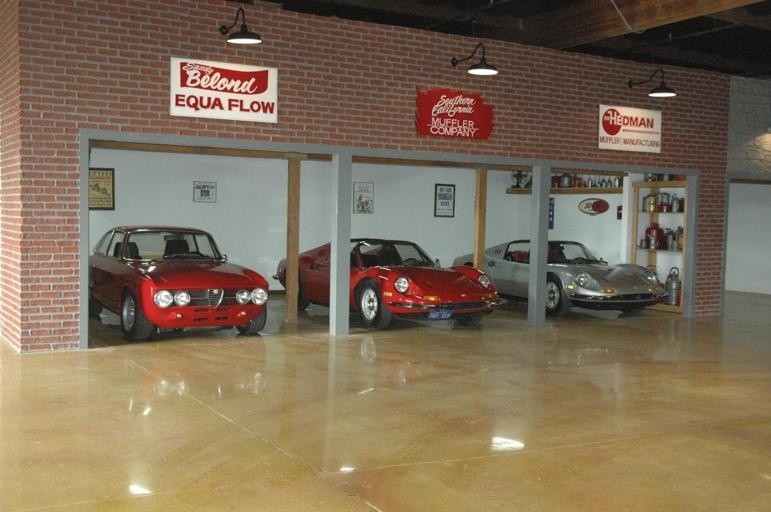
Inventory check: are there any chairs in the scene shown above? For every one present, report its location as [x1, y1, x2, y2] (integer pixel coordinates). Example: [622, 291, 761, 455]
[113, 241, 142, 259]
[379, 243, 403, 265]
[548, 243, 569, 262]
[163, 240, 191, 259]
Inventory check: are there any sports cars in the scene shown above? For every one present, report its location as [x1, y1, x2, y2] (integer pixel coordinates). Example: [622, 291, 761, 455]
[453, 239, 670, 318]
[273, 238, 509, 331]
[89, 225, 269, 341]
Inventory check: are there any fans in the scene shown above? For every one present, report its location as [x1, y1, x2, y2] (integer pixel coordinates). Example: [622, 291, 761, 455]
[511, 170, 532, 188]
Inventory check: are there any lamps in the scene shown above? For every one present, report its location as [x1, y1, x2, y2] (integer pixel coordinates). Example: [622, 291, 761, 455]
[451, 42, 499, 76]
[220, 7, 264, 46]
[629, 69, 678, 98]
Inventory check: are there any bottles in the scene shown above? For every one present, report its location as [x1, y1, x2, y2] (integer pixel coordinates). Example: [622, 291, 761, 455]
[582, 175, 622, 188]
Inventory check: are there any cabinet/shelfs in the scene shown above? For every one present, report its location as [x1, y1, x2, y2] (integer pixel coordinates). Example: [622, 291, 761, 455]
[633, 180, 688, 314]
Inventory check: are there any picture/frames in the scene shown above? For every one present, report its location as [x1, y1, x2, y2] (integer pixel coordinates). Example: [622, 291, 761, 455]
[89, 167, 115, 210]
[354, 182, 375, 214]
[435, 183, 455, 218]
[193, 181, 218, 203]
